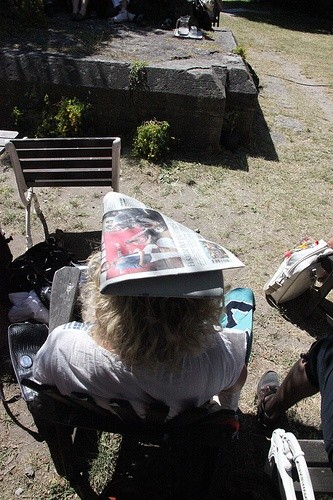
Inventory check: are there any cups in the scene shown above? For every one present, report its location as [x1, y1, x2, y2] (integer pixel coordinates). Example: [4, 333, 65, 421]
[191, 26, 197, 35]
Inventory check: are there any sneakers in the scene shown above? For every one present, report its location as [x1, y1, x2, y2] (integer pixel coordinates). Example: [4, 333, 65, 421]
[257, 370, 279, 420]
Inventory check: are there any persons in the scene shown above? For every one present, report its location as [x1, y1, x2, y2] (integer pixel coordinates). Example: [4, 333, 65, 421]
[34, 247, 248, 419]
[258, 339, 333, 470]
[73, 0, 222, 31]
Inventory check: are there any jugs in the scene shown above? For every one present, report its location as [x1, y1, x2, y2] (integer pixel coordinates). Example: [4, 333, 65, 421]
[176, 16, 190, 35]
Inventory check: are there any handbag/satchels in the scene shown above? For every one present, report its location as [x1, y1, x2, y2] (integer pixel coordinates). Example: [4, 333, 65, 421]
[263, 236, 333, 305]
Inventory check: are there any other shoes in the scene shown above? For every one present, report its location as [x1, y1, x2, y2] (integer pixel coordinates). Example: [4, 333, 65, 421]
[70, 12, 88, 21]
[113, 8, 135, 22]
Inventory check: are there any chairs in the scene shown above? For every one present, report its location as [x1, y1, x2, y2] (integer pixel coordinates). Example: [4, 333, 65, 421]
[8, 266, 255, 500]
[4, 137, 120, 252]
